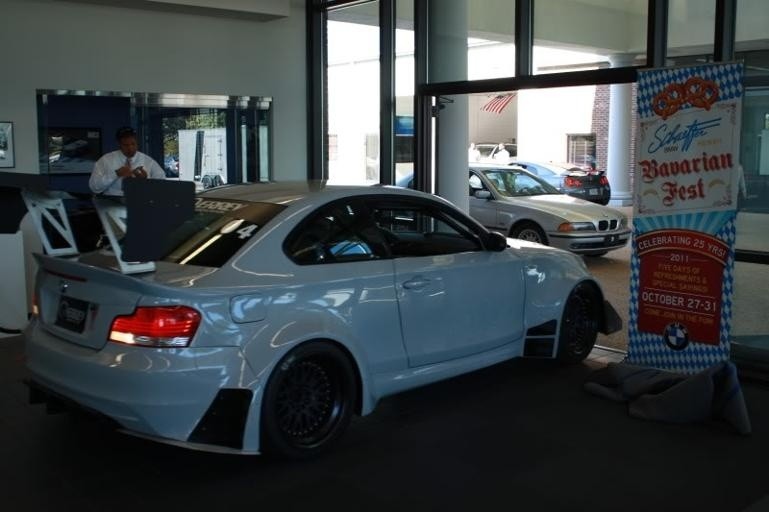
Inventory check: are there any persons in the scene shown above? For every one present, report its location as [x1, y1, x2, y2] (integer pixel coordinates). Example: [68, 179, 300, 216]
[493, 143, 510, 162]
[468, 142, 481, 162]
[88, 124, 167, 205]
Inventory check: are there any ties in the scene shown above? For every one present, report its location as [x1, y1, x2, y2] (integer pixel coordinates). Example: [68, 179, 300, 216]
[121, 158, 132, 192]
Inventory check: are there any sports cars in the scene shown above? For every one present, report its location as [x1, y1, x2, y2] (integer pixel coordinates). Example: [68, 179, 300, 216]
[20, 181, 618, 467]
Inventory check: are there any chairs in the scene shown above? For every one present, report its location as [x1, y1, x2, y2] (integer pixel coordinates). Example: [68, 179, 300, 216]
[301, 219, 395, 262]
[484, 178, 509, 199]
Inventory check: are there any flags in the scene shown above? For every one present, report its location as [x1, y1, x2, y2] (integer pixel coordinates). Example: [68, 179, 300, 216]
[479, 90, 518, 116]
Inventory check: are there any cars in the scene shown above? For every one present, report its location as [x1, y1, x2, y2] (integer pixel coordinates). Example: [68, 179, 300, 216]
[401, 156, 632, 260]
[163, 154, 180, 177]
